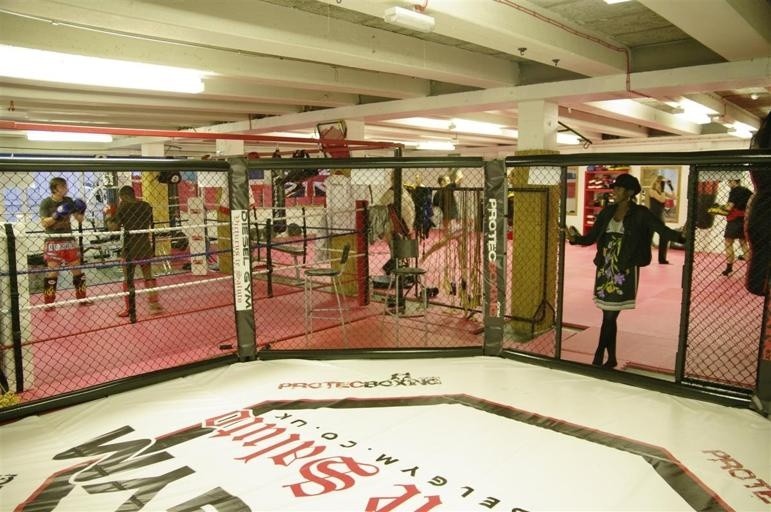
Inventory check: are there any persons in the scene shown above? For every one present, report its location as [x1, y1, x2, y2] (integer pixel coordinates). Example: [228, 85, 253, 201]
[706, 178, 755, 276]
[101, 185, 166, 317]
[744, 112, 769, 297]
[431, 170, 463, 232]
[650, 176, 677, 265]
[36, 177, 96, 313]
[567, 174, 690, 366]
[377, 169, 418, 288]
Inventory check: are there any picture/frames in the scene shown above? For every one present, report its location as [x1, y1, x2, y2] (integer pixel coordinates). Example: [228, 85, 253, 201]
[639, 165, 682, 223]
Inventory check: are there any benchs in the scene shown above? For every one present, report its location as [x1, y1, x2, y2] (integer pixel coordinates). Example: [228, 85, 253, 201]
[253, 240, 304, 282]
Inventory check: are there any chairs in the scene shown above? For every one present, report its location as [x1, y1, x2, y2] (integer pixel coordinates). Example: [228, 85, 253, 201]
[304, 243, 350, 344]
[380, 238, 428, 346]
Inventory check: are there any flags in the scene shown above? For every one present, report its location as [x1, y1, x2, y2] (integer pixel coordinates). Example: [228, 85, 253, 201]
[289, 166, 333, 200]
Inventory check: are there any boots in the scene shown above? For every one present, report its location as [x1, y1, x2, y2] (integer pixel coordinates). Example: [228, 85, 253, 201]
[118, 278, 137, 317]
[72, 272, 95, 306]
[42, 276, 60, 312]
[142, 278, 167, 313]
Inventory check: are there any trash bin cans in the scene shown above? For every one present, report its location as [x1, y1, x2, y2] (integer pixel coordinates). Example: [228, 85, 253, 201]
[28, 254, 45, 293]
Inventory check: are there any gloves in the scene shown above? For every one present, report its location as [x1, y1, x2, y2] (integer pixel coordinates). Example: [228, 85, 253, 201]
[101, 203, 117, 222]
[73, 199, 87, 216]
[53, 202, 76, 221]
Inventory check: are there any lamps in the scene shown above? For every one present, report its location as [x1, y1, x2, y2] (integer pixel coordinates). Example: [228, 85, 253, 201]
[416, 141, 456, 151]
[449, 118, 518, 138]
[26, 131, 114, 143]
[727, 120, 757, 141]
[384, 5, 436, 33]
[556, 133, 582, 145]
[672, 107, 711, 124]
[0, 45, 207, 96]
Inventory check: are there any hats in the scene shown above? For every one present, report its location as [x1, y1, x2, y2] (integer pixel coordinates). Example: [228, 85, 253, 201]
[610, 174, 642, 196]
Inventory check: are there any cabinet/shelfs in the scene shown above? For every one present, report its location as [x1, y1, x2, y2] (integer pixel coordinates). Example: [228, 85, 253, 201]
[583, 169, 629, 237]
